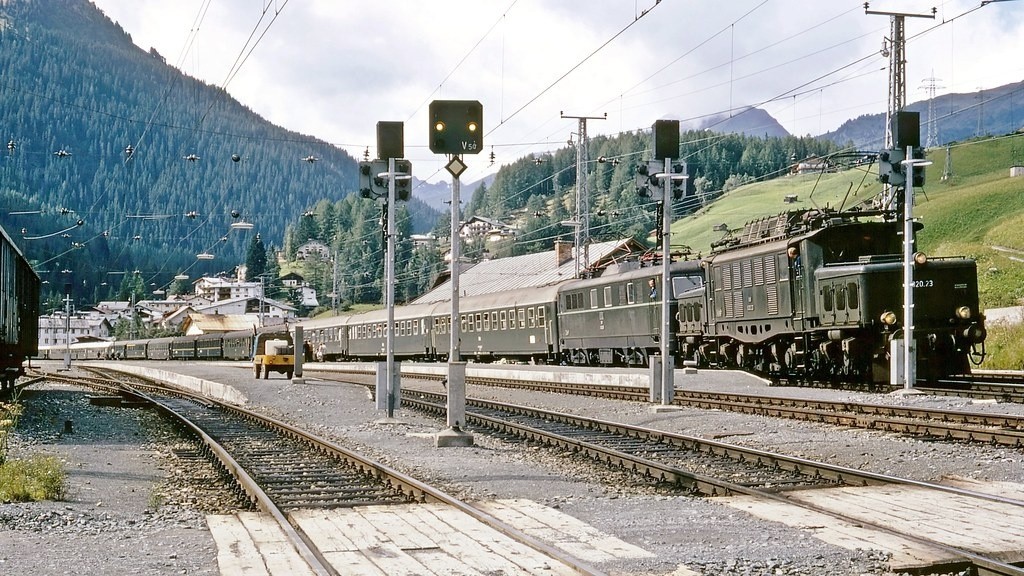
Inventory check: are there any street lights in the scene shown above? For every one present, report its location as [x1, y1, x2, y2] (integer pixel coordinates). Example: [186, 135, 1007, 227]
[889, 111, 932, 395]
[651, 118, 691, 411]
[429, 100, 484, 446]
[376, 122, 412, 418]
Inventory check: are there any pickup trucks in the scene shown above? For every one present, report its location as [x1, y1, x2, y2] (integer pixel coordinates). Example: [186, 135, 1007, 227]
[253, 332, 295, 379]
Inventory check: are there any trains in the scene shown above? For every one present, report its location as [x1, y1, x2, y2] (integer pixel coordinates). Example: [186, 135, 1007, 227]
[0, 224, 40, 390]
[39, 203, 989, 393]
